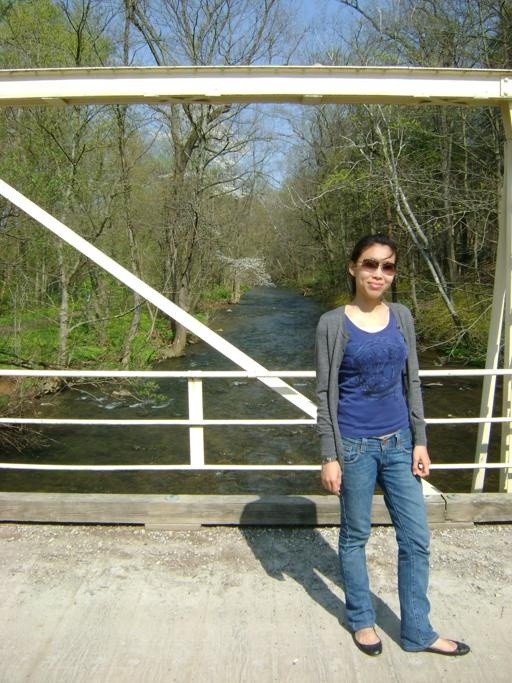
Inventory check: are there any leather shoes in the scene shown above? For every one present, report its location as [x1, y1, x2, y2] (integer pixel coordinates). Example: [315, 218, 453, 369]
[352, 626, 382, 655]
[423, 634, 470, 655]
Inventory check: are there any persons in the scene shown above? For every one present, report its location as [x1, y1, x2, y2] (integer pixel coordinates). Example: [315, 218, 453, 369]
[316, 234, 471, 657]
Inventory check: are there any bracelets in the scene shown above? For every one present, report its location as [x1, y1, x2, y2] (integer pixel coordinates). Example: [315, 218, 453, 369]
[321, 457, 334, 463]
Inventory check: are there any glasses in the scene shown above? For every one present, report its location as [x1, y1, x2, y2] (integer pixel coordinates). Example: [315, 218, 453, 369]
[356, 258, 399, 276]
